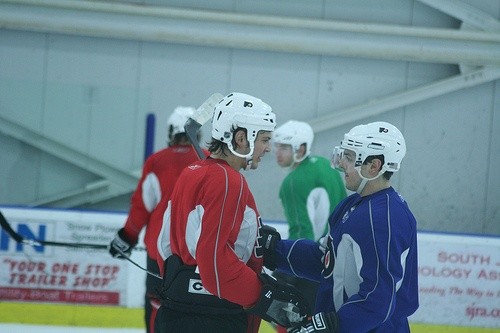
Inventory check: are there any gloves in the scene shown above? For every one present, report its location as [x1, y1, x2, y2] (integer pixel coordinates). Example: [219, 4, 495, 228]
[286, 311, 339, 333]
[109, 227, 138, 259]
[248, 273, 309, 327]
[260, 224, 281, 271]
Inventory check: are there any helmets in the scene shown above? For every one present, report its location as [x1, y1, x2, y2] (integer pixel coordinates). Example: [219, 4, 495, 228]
[272, 120, 313, 162]
[330, 121, 406, 180]
[167, 107, 202, 142]
[212, 92, 277, 158]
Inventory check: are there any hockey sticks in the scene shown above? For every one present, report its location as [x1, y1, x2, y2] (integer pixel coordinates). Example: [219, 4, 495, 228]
[0, 211, 147, 252]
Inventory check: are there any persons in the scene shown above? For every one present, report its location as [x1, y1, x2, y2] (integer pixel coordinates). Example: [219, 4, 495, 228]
[109, 106, 211, 333]
[154, 92, 309, 333]
[262, 121, 419, 333]
[273, 120, 348, 316]
